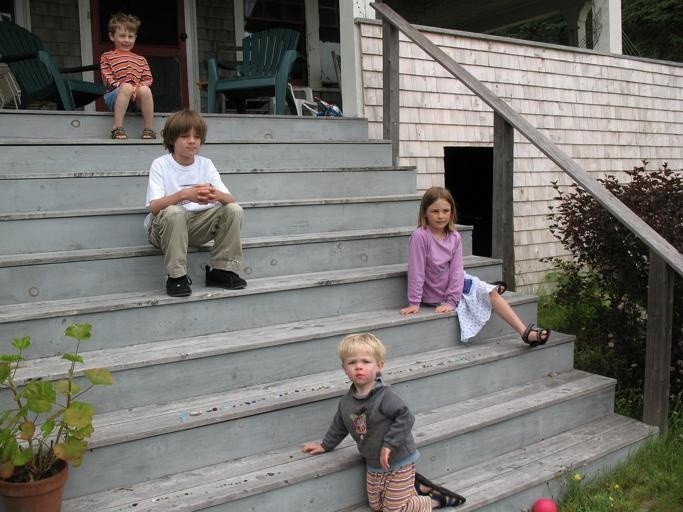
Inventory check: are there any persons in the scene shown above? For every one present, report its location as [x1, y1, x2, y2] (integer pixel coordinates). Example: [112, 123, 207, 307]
[301, 331, 466, 512]
[141, 108, 248, 298]
[99, 12, 156, 139]
[397, 185, 551, 350]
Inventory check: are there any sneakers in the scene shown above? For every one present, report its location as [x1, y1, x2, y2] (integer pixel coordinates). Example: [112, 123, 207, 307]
[205, 268, 247, 290]
[166, 277, 192, 297]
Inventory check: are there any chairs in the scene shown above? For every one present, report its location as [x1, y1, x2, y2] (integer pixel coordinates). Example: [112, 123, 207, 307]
[0, 16, 106, 111]
[205, 28, 300, 116]
[236, 66, 318, 116]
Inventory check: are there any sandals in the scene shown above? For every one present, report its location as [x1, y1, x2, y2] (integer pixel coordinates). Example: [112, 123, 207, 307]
[111, 126, 128, 139]
[414, 473, 433, 494]
[521, 323, 551, 347]
[428, 481, 466, 508]
[489, 281, 507, 296]
[141, 129, 158, 139]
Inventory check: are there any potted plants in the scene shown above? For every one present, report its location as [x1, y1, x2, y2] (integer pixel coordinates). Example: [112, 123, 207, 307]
[0, 323, 113, 512]
